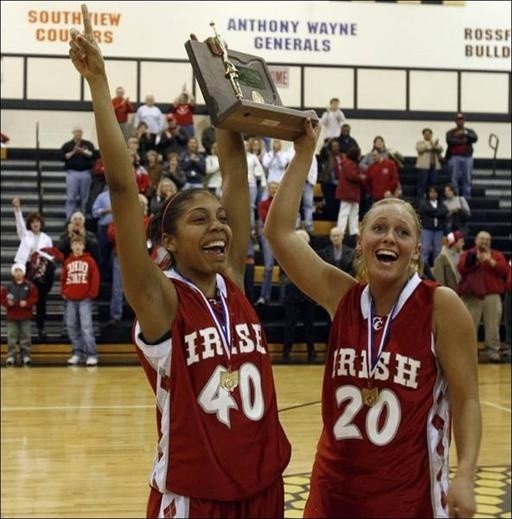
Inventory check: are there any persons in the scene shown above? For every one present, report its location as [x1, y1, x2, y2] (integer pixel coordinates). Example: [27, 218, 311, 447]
[0, 85, 289, 366]
[263, 111, 483, 517]
[65, 4, 292, 518]
[293, 95, 509, 364]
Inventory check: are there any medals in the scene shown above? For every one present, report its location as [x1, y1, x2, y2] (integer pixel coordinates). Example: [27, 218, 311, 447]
[218, 369, 240, 392]
[360, 386, 379, 408]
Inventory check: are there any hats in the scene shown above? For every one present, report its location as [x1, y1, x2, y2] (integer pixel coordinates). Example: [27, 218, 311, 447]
[455, 113, 464, 122]
[164, 111, 177, 122]
[446, 229, 465, 248]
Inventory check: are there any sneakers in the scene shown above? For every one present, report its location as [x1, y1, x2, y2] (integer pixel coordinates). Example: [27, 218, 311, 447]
[245, 295, 320, 363]
[486, 348, 511, 366]
[5, 319, 137, 367]
